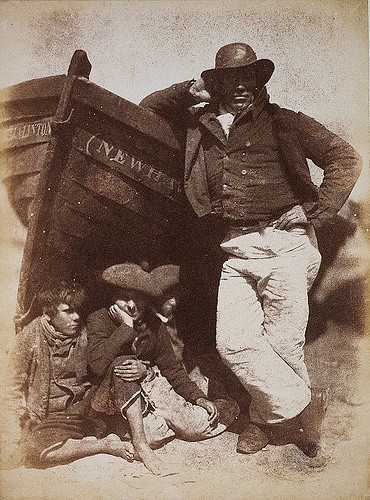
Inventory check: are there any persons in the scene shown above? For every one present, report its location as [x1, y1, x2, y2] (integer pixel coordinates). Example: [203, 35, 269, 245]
[0, 280, 174, 475]
[86, 262, 242, 449]
[150, 263, 209, 399]
[137, 42, 363, 457]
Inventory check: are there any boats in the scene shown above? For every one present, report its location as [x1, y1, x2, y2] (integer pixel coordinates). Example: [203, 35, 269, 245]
[1, 47, 357, 335]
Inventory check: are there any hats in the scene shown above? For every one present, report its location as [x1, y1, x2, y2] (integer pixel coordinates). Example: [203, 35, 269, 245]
[102, 263, 165, 299]
[148, 264, 180, 294]
[200, 43, 276, 89]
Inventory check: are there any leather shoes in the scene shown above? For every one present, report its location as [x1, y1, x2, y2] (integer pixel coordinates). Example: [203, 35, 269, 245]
[236, 422, 270, 455]
[299, 386, 330, 457]
